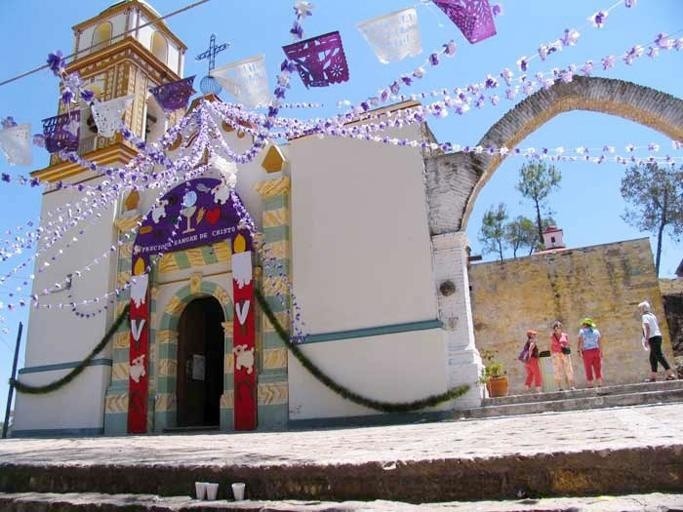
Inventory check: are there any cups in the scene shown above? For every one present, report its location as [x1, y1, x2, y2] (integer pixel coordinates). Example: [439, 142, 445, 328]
[232, 482, 245, 500]
[194, 482, 219, 501]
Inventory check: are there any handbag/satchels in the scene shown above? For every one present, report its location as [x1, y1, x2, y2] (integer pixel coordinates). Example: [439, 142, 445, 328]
[518, 349, 530, 362]
[562, 346, 571, 355]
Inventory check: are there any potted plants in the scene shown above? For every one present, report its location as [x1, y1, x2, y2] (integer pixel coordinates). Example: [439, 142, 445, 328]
[477, 345, 508, 397]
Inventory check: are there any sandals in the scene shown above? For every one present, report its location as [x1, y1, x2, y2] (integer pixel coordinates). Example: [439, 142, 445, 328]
[557, 386, 576, 392]
[644, 374, 676, 382]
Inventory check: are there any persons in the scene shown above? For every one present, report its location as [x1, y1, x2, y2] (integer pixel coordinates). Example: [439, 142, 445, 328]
[577, 318, 603, 387]
[638, 301, 672, 382]
[523, 330, 543, 393]
[548, 320, 575, 391]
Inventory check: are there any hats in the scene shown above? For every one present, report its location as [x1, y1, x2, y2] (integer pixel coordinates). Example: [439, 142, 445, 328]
[552, 321, 563, 329]
[527, 330, 537, 336]
[579, 317, 596, 327]
